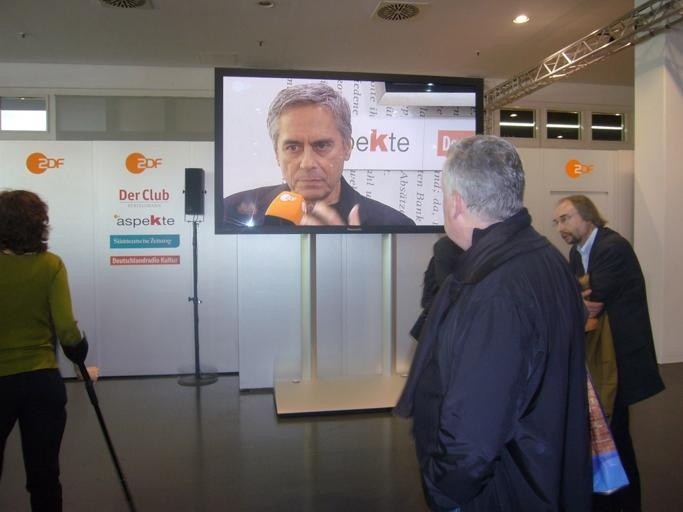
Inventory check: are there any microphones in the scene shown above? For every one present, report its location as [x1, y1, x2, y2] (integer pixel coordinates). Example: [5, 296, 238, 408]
[263, 191, 307, 226]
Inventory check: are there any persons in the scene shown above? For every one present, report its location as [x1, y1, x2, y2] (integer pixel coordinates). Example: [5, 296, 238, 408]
[1, 184, 100, 512]
[409, 234, 464, 343]
[403, 133, 594, 509]
[552, 197, 666, 512]
[218, 81, 420, 227]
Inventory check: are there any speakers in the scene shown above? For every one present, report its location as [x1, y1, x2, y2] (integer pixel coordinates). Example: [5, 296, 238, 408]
[185, 167, 205, 216]
